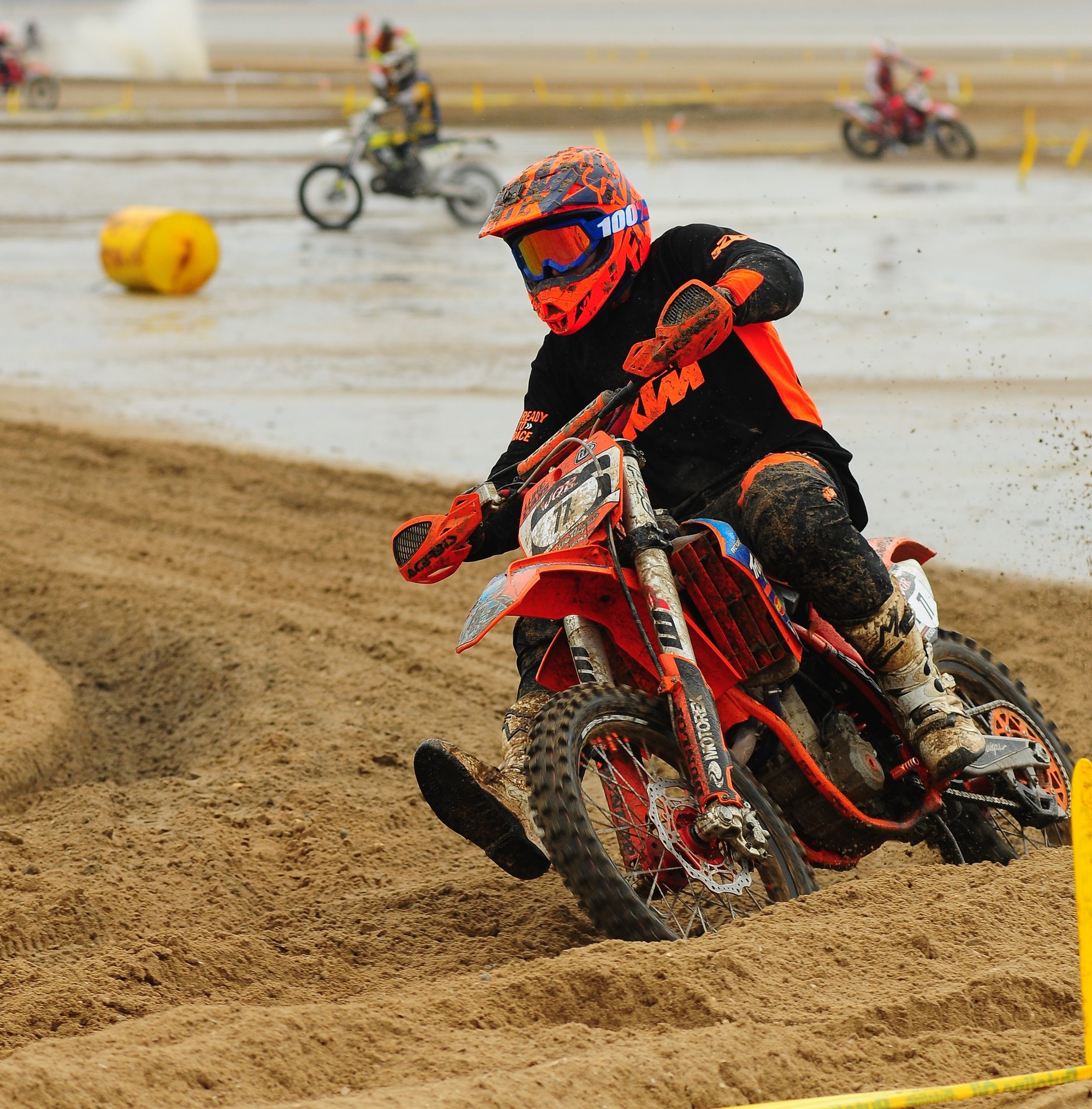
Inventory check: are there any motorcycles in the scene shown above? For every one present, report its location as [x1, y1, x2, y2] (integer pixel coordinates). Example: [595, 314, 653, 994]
[0, 25, 59, 115]
[831, 69, 980, 161]
[297, 97, 502, 229]
[392, 278, 1070, 941]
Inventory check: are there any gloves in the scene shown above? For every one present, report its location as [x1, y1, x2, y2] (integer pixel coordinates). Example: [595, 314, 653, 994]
[688, 286, 736, 350]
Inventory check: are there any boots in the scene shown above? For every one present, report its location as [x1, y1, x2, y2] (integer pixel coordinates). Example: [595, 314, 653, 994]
[414, 690, 589, 880]
[829, 573, 986, 782]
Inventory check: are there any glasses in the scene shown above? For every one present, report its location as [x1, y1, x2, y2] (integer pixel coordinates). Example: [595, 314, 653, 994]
[503, 218, 600, 280]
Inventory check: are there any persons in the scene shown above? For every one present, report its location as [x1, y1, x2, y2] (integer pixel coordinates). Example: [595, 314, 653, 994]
[865, 43, 929, 145]
[391, 149, 985, 881]
[362, 52, 439, 186]
[0, 26, 25, 90]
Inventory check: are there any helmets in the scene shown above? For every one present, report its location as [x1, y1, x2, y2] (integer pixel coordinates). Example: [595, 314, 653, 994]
[478, 145, 656, 333]
